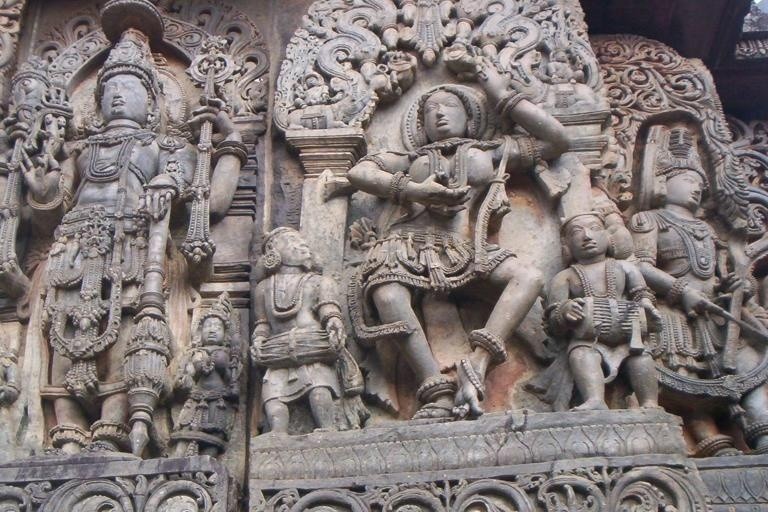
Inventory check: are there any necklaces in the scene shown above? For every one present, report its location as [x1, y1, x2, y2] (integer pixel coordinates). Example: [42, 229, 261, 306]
[570, 257, 616, 300]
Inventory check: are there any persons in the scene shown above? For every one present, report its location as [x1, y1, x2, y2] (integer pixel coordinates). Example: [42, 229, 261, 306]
[343, 61, 571, 422]
[248, 226, 346, 430]
[624, 159, 767, 454]
[172, 290, 243, 463]
[17, 24, 243, 458]
[548, 211, 659, 412]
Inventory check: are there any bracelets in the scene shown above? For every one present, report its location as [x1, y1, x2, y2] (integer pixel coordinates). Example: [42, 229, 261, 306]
[389, 170, 412, 205]
[28, 189, 65, 210]
[494, 88, 526, 116]
[666, 276, 687, 308]
[212, 140, 249, 170]
